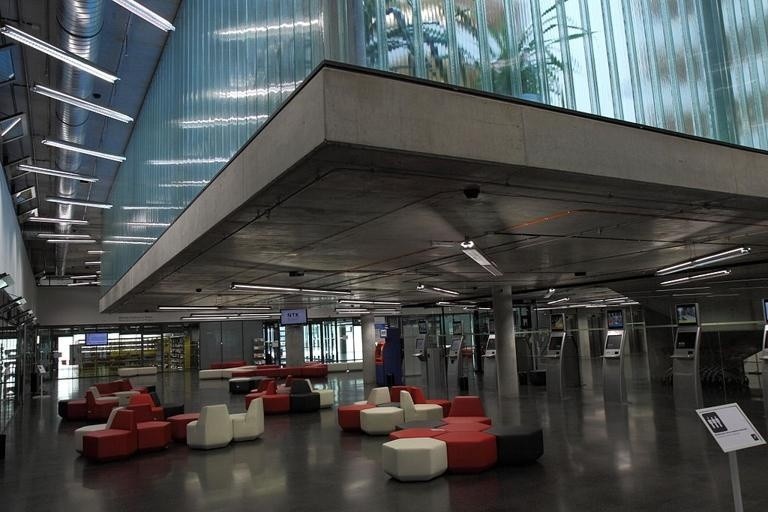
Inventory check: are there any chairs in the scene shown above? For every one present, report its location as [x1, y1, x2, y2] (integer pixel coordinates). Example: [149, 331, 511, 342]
[56, 356, 550, 479]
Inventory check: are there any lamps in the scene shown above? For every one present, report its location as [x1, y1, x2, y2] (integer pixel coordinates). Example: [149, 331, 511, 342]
[156, 282, 641, 320]
[1, 272, 38, 330]
[460, 235, 505, 279]
[653, 247, 751, 285]
[1, 0, 177, 290]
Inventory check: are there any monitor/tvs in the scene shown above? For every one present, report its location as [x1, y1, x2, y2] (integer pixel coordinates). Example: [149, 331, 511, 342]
[606, 335, 622, 350]
[765, 330, 768, 350]
[280, 308, 307, 326]
[85, 332, 108, 345]
[677, 331, 696, 349]
[418, 321, 428, 335]
[416, 338, 425, 351]
[675, 303, 700, 326]
[451, 338, 461, 350]
[486, 338, 496, 351]
[488, 319, 495, 334]
[453, 321, 463, 335]
[606, 309, 625, 329]
[762, 298, 768, 325]
[549, 336, 562, 350]
[549, 313, 566, 332]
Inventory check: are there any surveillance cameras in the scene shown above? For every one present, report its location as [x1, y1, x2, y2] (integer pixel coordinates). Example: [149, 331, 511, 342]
[464, 185, 480, 198]
